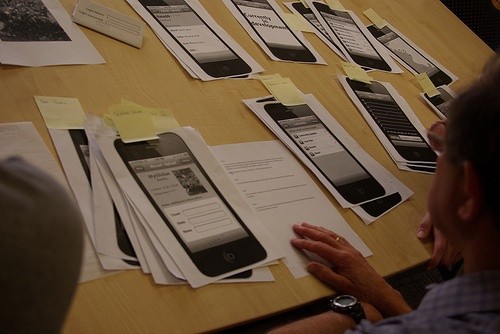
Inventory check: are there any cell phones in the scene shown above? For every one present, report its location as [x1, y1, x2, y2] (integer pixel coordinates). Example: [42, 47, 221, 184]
[69, 0, 455, 281]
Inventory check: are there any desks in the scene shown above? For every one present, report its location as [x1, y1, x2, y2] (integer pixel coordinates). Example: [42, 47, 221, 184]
[0, 0, 500, 334]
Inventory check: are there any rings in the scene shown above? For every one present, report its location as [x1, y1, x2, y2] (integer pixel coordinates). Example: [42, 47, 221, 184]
[335, 236, 340, 242]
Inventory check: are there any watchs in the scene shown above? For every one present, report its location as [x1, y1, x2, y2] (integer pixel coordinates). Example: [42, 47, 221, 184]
[329, 293, 367, 324]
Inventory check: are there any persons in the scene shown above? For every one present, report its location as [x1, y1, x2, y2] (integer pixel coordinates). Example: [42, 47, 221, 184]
[417, 52, 500, 271]
[263, 67, 500, 334]
[1, 151, 85, 334]
